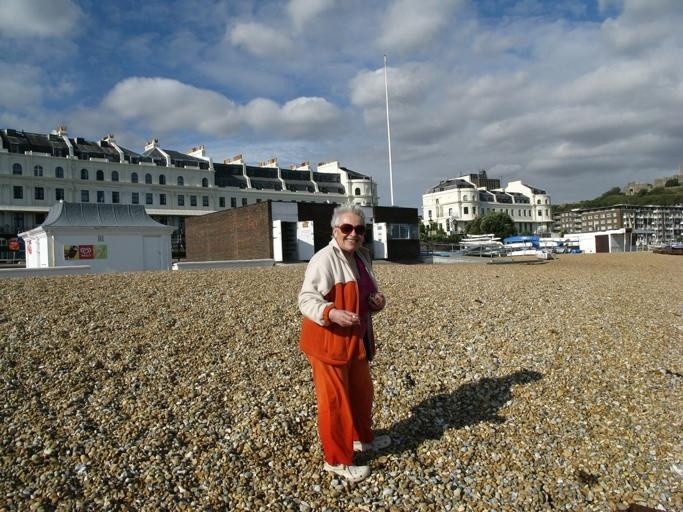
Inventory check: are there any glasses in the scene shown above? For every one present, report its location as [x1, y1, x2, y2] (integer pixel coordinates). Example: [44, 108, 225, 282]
[335, 224, 366, 235]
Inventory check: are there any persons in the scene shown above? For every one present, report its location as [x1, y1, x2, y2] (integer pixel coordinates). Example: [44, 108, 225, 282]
[297, 203, 391, 482]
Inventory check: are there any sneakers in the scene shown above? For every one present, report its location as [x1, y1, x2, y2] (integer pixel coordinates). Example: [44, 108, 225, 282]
[353, 435, 391, 452]
[324, 461, 370, 482]
